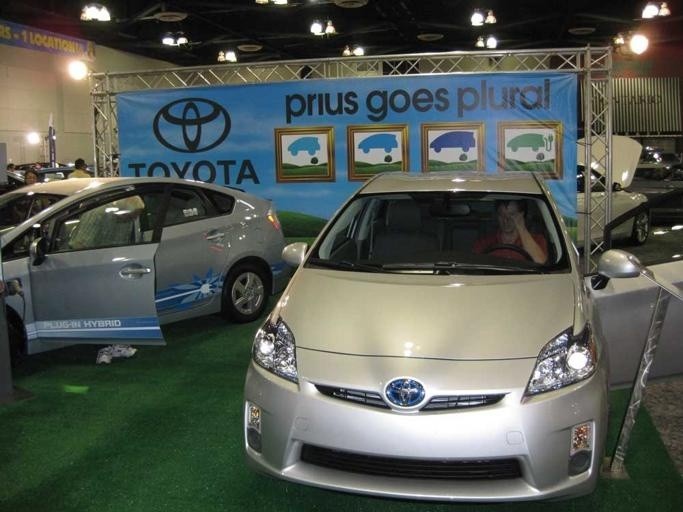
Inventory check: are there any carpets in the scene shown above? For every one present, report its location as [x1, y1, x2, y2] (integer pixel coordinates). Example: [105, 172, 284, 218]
[1, 272, 682, 511]
[577, 216, 682, 278]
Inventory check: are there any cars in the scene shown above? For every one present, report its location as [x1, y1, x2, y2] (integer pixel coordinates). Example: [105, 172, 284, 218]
[0, 176, 288, 372]
[7, 162, 105, 189]
[627, 147, 683, 224]
[575, 134, 649, 252]
[240, 168, 683, 504]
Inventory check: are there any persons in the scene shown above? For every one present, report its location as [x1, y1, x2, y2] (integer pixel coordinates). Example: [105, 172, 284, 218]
[7, 158, 91, 179]
[470, 199, 551, 265]
[13, 170, 50, 224]
[68, 195, 146, 366]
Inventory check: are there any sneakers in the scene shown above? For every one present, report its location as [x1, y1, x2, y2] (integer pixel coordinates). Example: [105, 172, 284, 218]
[111, 343, 139, 359]
[95, 343, 115, 366]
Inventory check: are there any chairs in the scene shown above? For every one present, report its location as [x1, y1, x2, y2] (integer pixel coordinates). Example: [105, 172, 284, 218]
[369, 198, 440, 260]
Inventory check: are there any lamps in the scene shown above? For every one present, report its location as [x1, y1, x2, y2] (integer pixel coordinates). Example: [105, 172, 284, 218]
[219, 48, 237, 62]
[343, 43, 365, 55]
[471, 7, 498, 26]
[640, 1, 670, 18]
[312, 19, 336, 35]
[80, 2, 111, 21]
[161, 27, 187, 47]
[476, 34, 497, 49]
